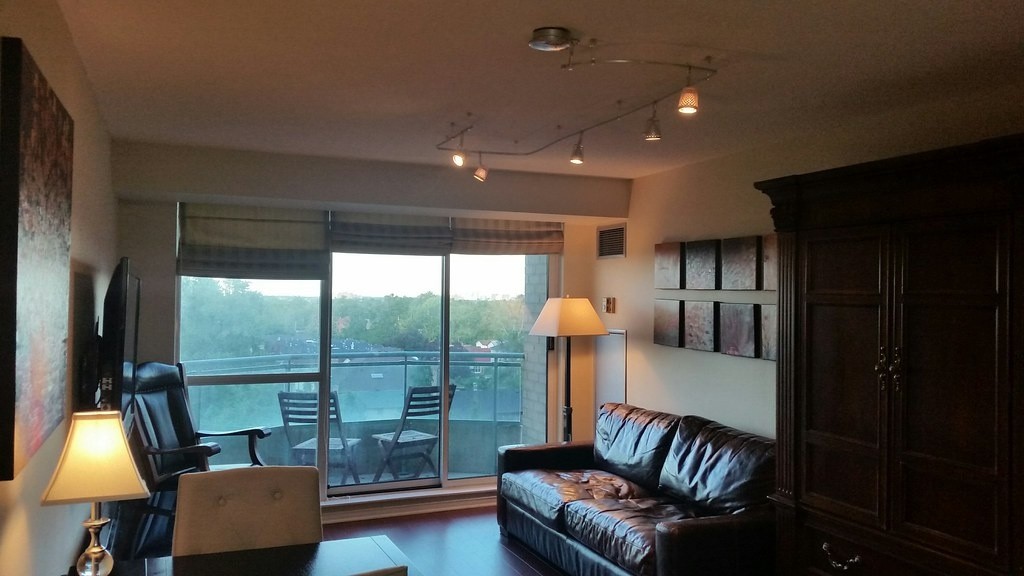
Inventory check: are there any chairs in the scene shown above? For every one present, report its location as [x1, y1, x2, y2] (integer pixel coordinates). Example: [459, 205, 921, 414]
[277, 390, 362, 486]
[171, 466, 322, 557]
[132, 361, 273, 492]
[370, 384, 457, 483]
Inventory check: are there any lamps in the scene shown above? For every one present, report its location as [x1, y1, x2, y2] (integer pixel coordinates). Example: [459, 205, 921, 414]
[644, 101, 661, 140]
[473, 151, 489, 182]
[678, 67, 699, 114]
[451, 133, 468, 168]
[570, 132, 584, 164]
[528, 28, 571, 51]
[528, 294, 609, 441]
[39, 410, 152, 576]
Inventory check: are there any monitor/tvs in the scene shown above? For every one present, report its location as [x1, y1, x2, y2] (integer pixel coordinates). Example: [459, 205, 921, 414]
[103, 256, 141, 440]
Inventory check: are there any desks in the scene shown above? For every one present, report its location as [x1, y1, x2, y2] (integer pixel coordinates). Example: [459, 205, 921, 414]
[68, 536, 426, 576]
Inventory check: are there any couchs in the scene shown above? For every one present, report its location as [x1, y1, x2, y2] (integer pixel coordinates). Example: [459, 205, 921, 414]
[497, 402, 776, 576]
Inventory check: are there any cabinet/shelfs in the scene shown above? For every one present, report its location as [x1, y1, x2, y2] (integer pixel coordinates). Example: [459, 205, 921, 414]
[751, 132, 1024, 575]
